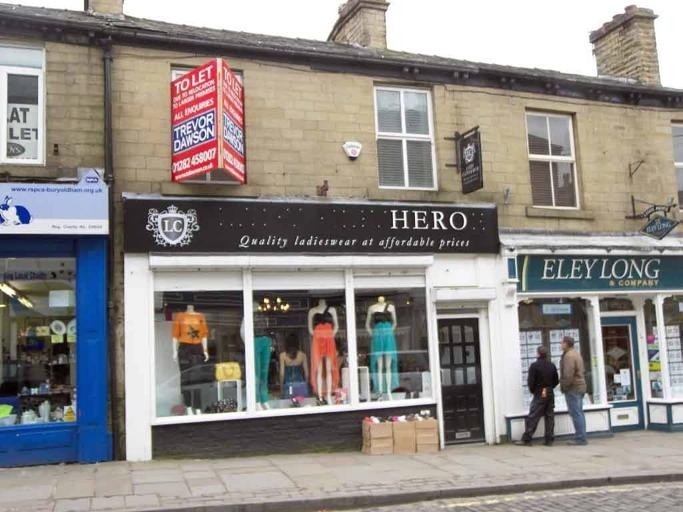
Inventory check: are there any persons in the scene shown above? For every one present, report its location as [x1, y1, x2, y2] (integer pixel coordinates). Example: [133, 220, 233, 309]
[305, 299, 347, 407]
[278, 335, 309, 404]
[557, 333, 590, 442]
[510, 346, 559, 448]
[238, 305, 274, 414]
[165, 304, 212, 418]
[362, 295, 397, 401]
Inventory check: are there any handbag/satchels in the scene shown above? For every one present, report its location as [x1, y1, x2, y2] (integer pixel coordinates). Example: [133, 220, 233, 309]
[215, 362, 242, 380]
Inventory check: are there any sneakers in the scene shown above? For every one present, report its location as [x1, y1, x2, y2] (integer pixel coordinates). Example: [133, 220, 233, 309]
[567, 438, 587, 445]
[543, 441, 552, 445]
[514, 440, 530, 445]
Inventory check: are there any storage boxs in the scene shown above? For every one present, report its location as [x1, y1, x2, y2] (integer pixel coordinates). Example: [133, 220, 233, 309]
[361, 417, 438, 456]
